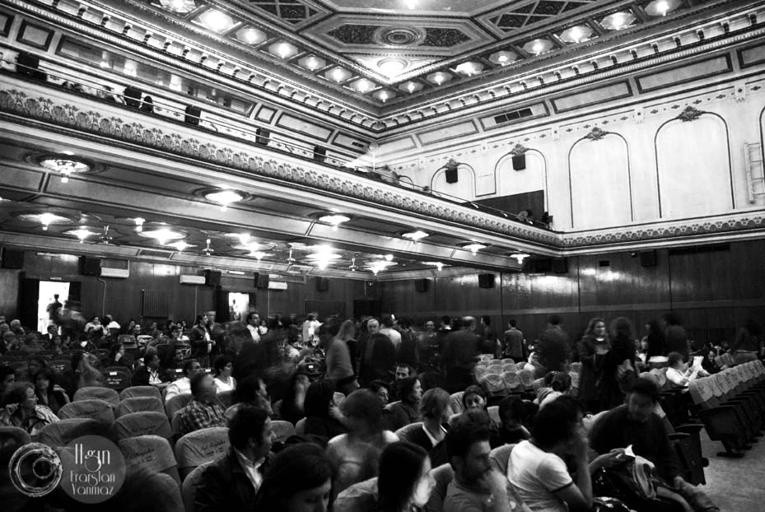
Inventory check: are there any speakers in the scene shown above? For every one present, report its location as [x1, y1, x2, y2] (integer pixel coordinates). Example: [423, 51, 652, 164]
[640, 252, 657, 266]
[318, 277, 327, 292]
[415, 279, 427, 293]
[479, 275, 493, 288]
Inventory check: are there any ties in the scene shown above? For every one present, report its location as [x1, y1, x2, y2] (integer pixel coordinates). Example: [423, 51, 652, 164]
[257, 327, 263, 337]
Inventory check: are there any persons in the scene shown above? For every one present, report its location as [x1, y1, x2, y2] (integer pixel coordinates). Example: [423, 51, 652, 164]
[0, 293, 765, 512]
